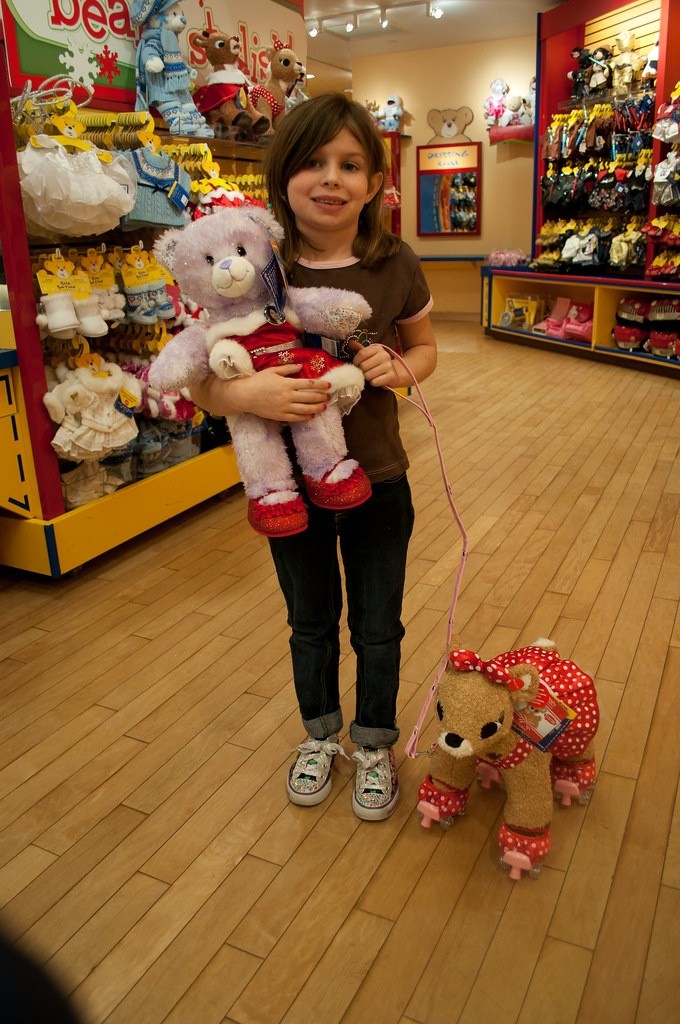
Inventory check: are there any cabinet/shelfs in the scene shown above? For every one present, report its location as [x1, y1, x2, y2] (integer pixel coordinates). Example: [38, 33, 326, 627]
[484, 268, 680, 371]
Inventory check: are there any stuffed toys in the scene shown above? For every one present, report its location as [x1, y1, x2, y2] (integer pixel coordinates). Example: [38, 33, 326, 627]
[418, 638, 599, 879]
[484, 79, 509, 131]
[567, 45, 613, 103]
[189, 31, 269, 142]
[150, 204, 372, 537]
[501, 75, 535, 127]
[250, 43, 307, 147]
[381, 96, 404, 133]
[129, 0, 215, 139]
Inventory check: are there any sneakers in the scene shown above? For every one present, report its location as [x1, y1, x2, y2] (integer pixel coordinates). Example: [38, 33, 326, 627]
[285, 730, 399, 820]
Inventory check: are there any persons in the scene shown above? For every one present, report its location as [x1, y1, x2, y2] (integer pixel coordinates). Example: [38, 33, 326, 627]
[188, 96, 438, 822]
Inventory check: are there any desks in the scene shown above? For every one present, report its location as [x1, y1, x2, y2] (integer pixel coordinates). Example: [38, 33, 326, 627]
[419, 254, 488, 325]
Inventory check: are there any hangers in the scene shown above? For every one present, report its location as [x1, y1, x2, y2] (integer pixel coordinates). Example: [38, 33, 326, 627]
[13, 94, 268, 378]
[529, 82, 680, 268]
